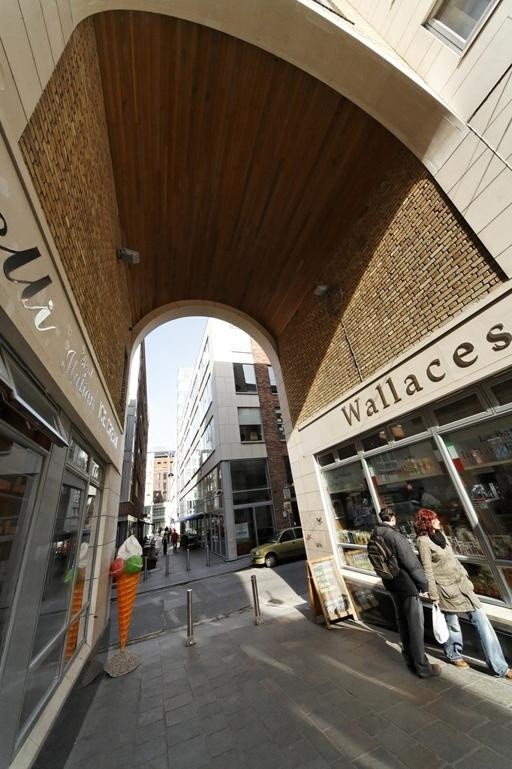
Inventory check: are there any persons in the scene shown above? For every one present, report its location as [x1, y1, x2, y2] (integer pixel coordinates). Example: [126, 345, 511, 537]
[370, 508, 441, 679]
[158, 526, 178, 555]
[414, 508, 512, 678]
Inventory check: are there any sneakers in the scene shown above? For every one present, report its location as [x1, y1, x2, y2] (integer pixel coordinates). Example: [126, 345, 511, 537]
[418, 664, 442, 678]
[505, 668, 512, 678]
[452, 659, 469, 668]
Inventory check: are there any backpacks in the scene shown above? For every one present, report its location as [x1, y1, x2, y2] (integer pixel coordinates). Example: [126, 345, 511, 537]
[367, 528, 399, 579]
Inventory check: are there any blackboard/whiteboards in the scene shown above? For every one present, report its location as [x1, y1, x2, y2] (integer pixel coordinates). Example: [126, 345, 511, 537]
[310, 556, 354, 622]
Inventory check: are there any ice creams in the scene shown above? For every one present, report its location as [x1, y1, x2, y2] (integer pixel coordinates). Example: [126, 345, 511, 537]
[111, 535, 143, 652]
[63, 542, 88, 658]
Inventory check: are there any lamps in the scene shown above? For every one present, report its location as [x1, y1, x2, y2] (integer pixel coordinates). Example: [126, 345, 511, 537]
[116, 246, 142, 264]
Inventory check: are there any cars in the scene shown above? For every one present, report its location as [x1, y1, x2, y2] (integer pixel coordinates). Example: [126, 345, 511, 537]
[250, 526, 306, 568]
